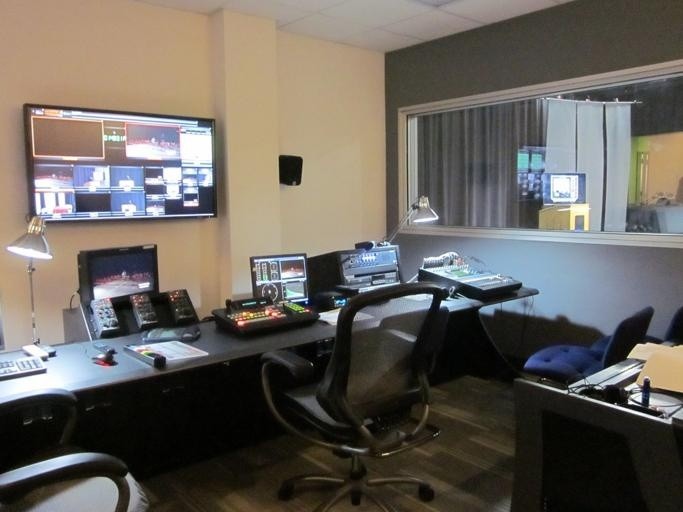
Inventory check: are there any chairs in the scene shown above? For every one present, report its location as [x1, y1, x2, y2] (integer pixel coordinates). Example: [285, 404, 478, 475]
[254, 281, 448, 511]
[0, 388, 150, 512]
[524, 306, 654, 389]
[590, 306, 683, 362]
[656, 205, 683, 234]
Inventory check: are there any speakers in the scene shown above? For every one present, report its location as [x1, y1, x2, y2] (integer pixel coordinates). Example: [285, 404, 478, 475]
[279, 155, 303, 186]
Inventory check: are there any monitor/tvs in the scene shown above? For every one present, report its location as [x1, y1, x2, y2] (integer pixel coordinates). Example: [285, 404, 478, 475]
[250, 253, 313, 307]
[23, 103, 218, 224]
[541, 172, 587, 205]
[78, 244, 159, 308]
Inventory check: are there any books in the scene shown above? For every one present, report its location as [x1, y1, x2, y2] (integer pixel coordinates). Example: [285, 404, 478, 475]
[625, 341, 683, 395]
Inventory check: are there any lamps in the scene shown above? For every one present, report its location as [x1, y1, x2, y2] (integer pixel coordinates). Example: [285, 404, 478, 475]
[383, 196, 439, 243]
[5, 216, 56, 356]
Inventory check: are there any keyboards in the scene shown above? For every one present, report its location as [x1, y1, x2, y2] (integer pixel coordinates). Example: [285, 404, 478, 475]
[0, 356, 47, 379]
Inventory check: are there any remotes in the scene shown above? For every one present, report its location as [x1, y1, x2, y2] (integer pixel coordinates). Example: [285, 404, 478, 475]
[22, 344, 49, 361]
[38, 344, 56, 357]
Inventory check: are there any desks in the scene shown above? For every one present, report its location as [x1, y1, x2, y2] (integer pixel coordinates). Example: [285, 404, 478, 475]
[0, 285, 539, 480]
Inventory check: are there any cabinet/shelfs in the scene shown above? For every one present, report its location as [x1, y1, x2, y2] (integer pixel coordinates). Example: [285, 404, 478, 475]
[538, 203, 590, 230]
[510, 378, 683, 511]
